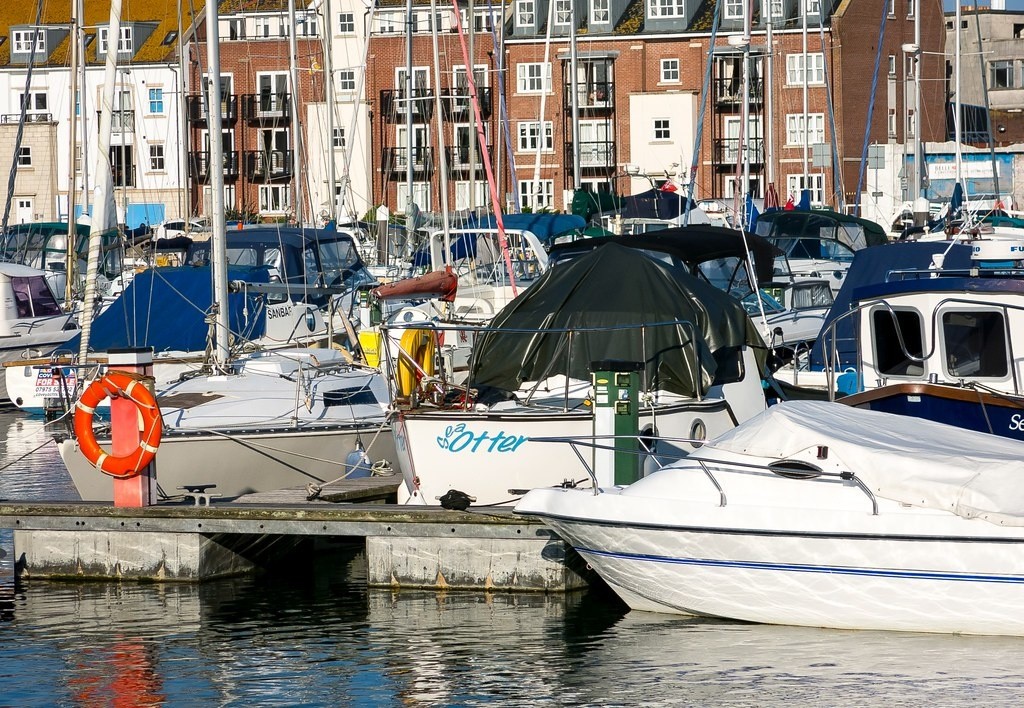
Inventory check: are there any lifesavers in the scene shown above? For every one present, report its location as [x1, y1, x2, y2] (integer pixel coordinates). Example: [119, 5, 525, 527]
[72, 371, 165, 483]
[396, 321, 437, 405]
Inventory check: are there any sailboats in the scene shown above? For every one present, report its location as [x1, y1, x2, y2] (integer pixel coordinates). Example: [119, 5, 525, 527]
[3, 1, 1024, 508]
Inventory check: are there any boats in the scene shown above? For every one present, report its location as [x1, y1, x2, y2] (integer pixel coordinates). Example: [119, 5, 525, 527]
[772, 286, 1024, 441]
[389, 246, 785, 510]
[515, 396, 1024, 643]
